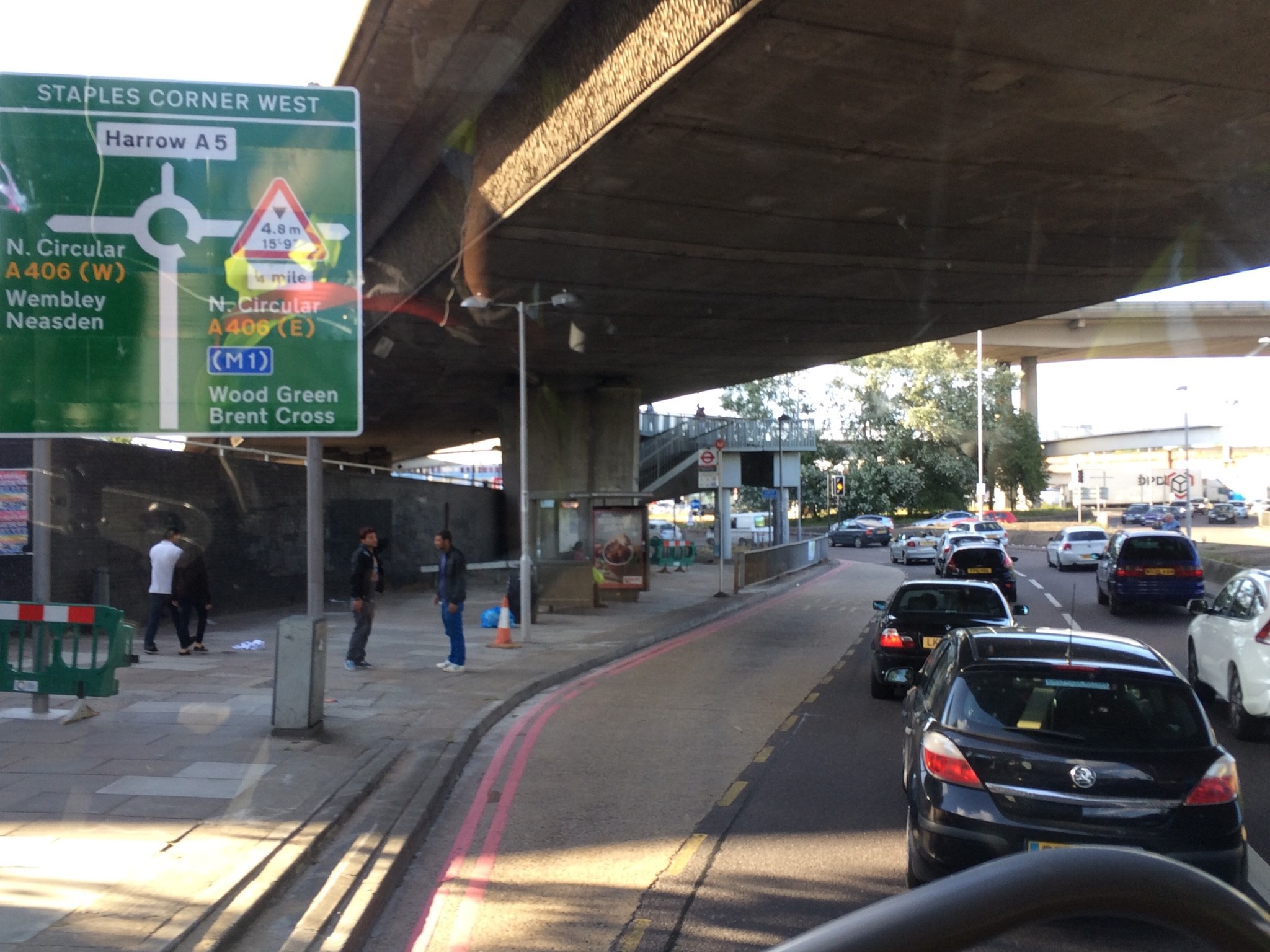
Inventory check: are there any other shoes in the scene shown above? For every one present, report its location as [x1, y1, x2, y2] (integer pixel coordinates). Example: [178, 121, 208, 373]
[356, 660, 373, 667]
[144, 648, 159, 654]
[179, 650, 191, 655]
[194, 645, 208, 651]
[345, 660, 355, 671]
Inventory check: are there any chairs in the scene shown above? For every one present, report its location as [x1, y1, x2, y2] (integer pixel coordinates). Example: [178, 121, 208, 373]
[908, 596, 930, 610]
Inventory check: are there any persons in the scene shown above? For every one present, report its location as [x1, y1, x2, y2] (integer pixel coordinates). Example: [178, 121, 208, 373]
[170, 535, 212, 654]
[431, 530, 467, 674]
[344, 527, 382, 671]
[1159, 512, 1181, 531]
[142, 527, 194, 656]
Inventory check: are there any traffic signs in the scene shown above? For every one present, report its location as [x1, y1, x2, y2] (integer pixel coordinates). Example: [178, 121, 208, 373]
[1, 69, 366, 441]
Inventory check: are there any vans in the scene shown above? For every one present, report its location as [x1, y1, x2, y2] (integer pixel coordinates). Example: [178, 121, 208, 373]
[704, 511, 775, 551]
[1090, 529, 1206, 618]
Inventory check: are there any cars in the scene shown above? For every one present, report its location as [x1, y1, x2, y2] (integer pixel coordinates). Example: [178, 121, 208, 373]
[631, 519, 682, 543]
[1120, 498, 1270, 528]
[825, 511, 1019, 603]
[867, 576, 1029, 701]
[1045, 525, 1110, 572]
[1184, 566, 1270, 741]
[880, 624, 1251, 897]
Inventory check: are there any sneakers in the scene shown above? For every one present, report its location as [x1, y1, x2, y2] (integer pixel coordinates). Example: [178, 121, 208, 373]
[436, 660, 452, 668]
[443, 663, 464, 672]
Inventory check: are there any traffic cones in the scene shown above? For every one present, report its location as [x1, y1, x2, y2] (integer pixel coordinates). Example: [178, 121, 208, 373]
[485, 594, 523, 650]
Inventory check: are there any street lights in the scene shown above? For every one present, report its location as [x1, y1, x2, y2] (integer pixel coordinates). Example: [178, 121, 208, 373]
[1175, 386, 1193, 539]
[778, 414, 795, 544]
[459, 291, 580, 646]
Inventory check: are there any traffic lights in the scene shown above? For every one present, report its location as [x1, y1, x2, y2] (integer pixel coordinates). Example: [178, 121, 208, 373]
[834, 475, 846, 497]
